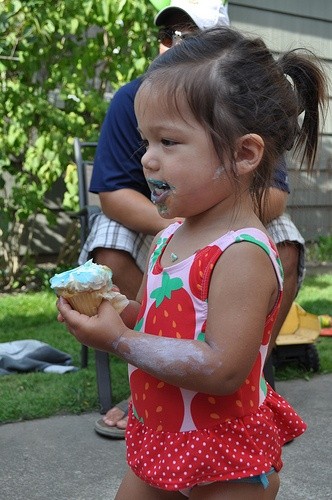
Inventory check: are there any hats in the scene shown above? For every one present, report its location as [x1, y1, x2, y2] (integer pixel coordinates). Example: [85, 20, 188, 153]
[154, 0, 231, 33]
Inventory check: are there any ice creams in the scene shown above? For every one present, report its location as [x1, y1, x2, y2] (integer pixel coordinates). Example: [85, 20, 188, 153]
[50, 258, 113, 317]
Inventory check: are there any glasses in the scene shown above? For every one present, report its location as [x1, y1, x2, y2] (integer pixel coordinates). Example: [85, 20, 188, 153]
[158, 27, 185, 48]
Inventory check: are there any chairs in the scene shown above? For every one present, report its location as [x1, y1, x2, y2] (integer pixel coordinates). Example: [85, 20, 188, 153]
[73, 138, 113, 416]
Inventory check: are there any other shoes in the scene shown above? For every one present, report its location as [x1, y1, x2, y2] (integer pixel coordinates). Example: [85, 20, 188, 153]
[95, 398, 132, 438]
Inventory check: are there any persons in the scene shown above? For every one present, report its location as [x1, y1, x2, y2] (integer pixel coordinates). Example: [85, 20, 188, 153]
[74, 6, 307, 440]
[56, 20, 331, 500]
